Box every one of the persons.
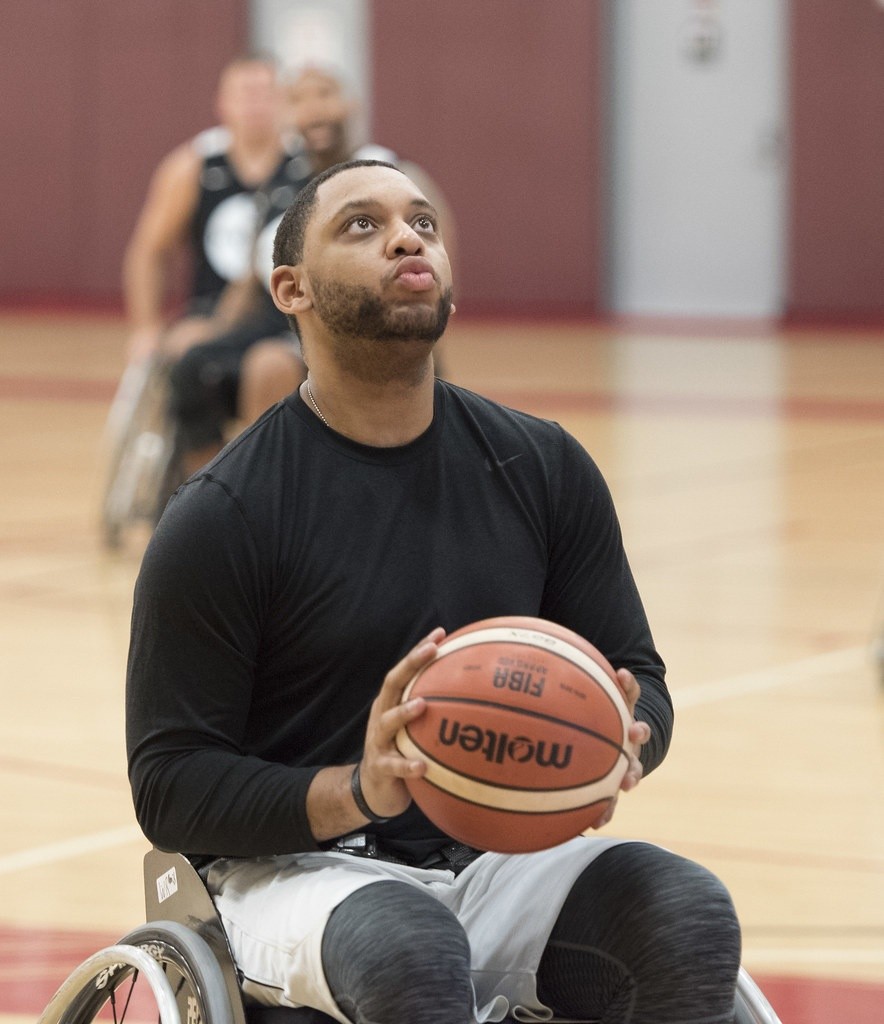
[123,154,757,1023]
[123,42,363,500]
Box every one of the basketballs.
[394,614,634,855]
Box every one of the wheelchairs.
[29,848,783,1023]
[95,352,195,556]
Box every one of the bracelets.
[349,764,396,826]
